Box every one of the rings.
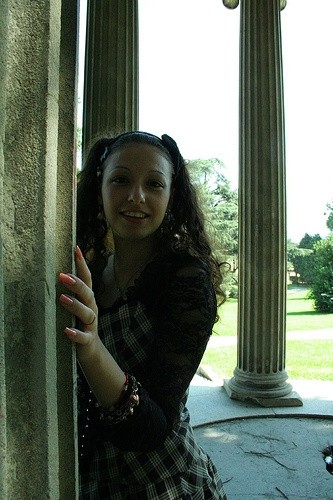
[84,313,96,326]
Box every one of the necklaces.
[113,252,154,300]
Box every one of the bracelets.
[96,372,140,424]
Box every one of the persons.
[59,131,228,500]
